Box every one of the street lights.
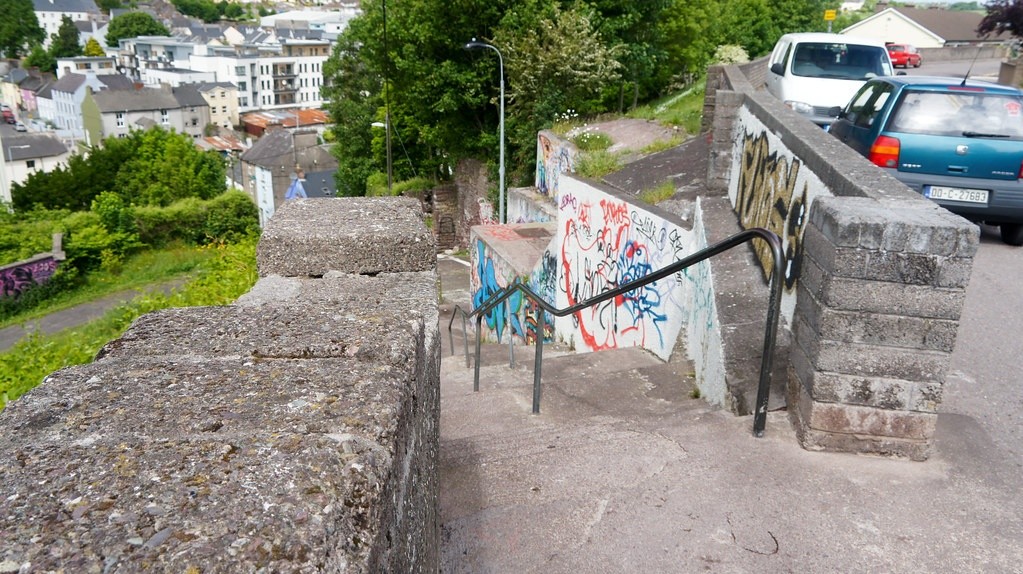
[466,42,505,224]
[8,144,31,190]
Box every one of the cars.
[885,43,922,68]
[1,104,26,132]
[828,76,1023,246]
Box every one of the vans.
[763,32,897,132]
